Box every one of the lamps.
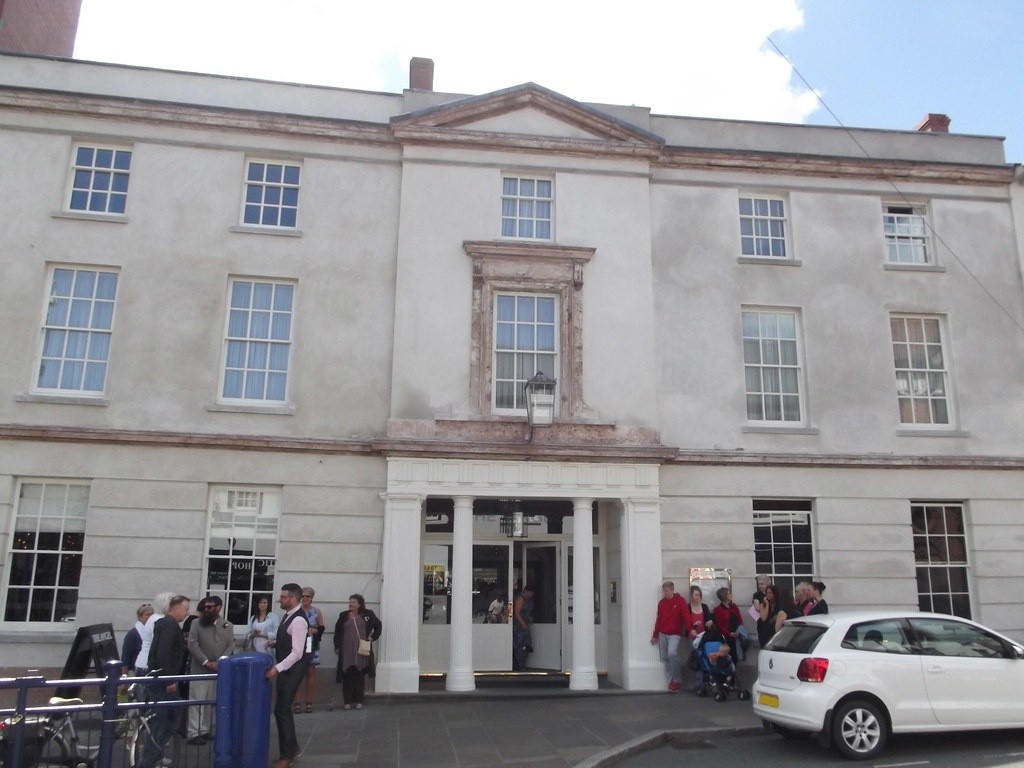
[524,370,557,442]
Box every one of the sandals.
[305,703,312,713]
[293,702,302,714]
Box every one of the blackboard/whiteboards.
[52,624,123,720]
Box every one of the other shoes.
[292,749,302,759]
[356,703,362,709]
[136,737,144,749]
[669,678,680,690]
[188,736,207,745]
[201,734,215,740]
[176,729,187,738]
[155,757,172,765]
[124,736,133,751]
[270,757,295,768]
[164,742,169,747]
[345,704,351,709]
[667,687,677,695]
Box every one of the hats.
[812,581,826,597]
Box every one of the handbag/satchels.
[686,650,699,671]
[306,628,312,653]
[520,633,534,653]
[748,604,761,622]
[358,639,371,656]
[244,632,256,652]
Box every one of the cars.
[748,613,1024,757]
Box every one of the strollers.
[694,624,754,705]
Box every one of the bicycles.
[0,669,181,768]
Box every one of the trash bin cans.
[214,651,274,768]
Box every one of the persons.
[121,604,154,751]
[135,592,191,768]
[188,596,236,745]
[712,587,743,658]
[513,585,536,670]
[650,581,692,692]
[246,596,279,655]
[334,594,382,712]
[488,593,505,623]
[294,587,325,713]
[686,585,713,638]
[710,644,730,660]
[753,575,829,649]
[266,583,310,768]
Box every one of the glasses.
[143,603,151,608]
[202,604,216,610]
[279,595,294,599]
[302,595,310,599]
